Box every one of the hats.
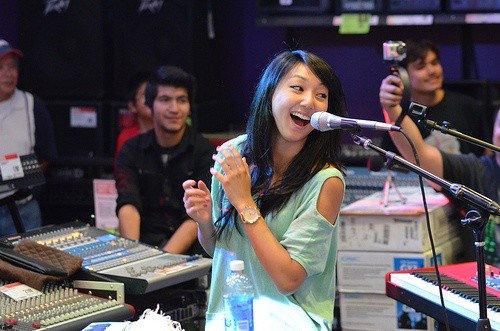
[0,40,22,64]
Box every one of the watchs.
[239,206,264,225]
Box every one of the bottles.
[222,259,255,331]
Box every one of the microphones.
[310,111,401,132]
[367,156,410,174]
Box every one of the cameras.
[383,40,407,61]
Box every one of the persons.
[114,64,223,260]
[379,72,500,270]
[112,72,156,178]
[183,49,347,331]
[377,38,496,159]
[1,39,59,240]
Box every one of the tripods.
[379,176,407,208]
